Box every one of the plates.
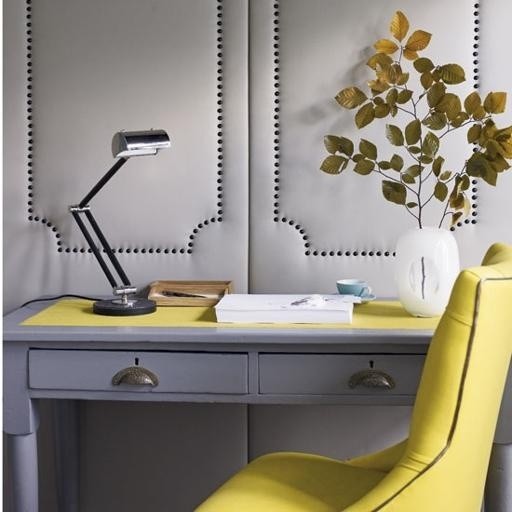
[359,294,377,302]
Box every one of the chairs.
[197,242,512,512]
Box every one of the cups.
[336,278,371,298]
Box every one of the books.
[213,293,354,324]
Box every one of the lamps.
[68,129,171,316]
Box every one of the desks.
[3,298,512,511]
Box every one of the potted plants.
[320,9,512,320]
